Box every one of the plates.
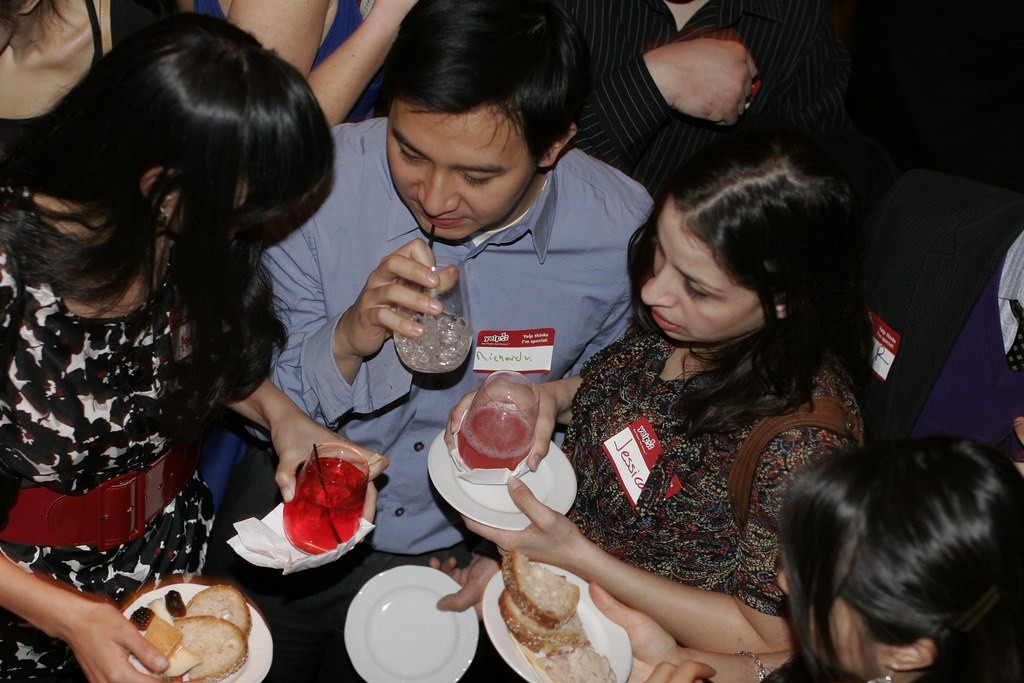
[482,562,633,683]
[122,583,273,683]
[344,565,479,683]
[427,429,577,531]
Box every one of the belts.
[1,426,201,551]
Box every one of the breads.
[174,618,248,683]
[499,550,579,630]
[513,631,592,655]
[183,585,250,637]
[498,587,584,642]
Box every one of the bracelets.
[734,650,765,682]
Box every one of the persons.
[442,125,864,683]
[1,15,389,683]
[220,0,659,682]
[589,444,1024,683]
[0,0,1023,438]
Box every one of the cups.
[392,257,473,373]
[457,371,540,471]
[283,442,370,556]
